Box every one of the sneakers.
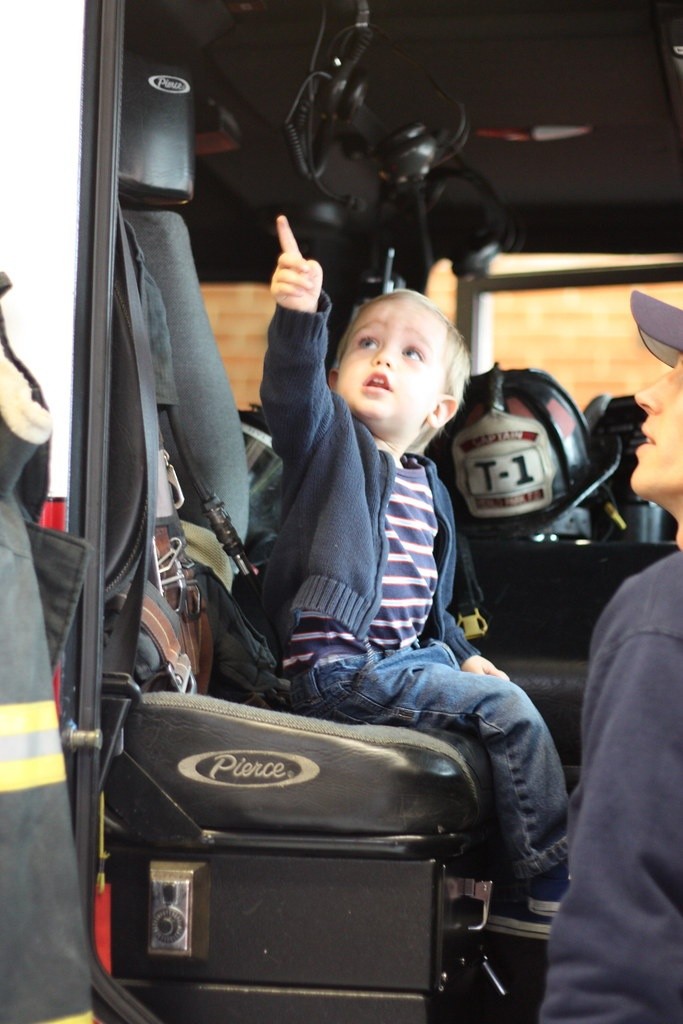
[486,901,554,939]
[529,875,571,918]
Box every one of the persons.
[259,215,571,918]
[534,290,683,1024]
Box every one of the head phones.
[330,25,469,178]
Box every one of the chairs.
[103,194,496,857]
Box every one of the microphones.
[309,94,366,214]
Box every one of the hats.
[630,290,683,368]
